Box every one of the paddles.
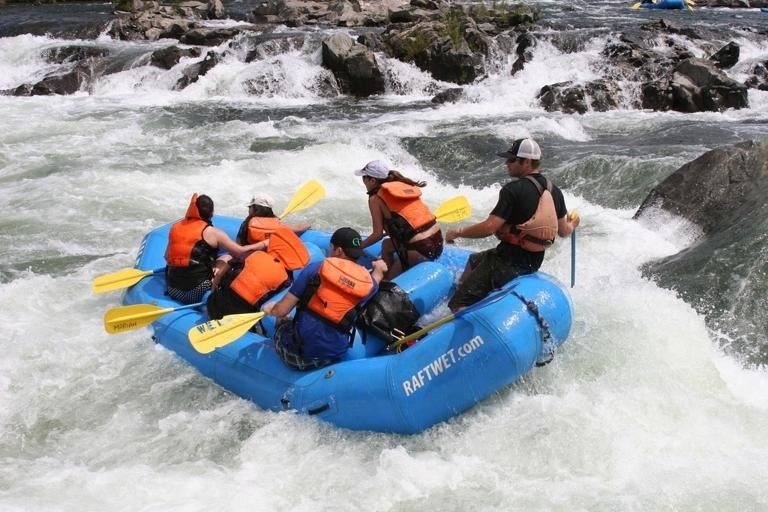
[188,268,374,354]
[385,283,517,353]
[382,196,472,237]
[92,267,166,295]
[105,300,208,333]
[278,179,325,219]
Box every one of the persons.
[234,196,311,270]
[163,194,273,309]
[355,160,444,281]
[205,247,292,322]
[444,134,582,314]
[262,225,389,371]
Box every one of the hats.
[245,197,273,209]
[354,160,390,180]
[330,227,366,258]
[495,138,544,161]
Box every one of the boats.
[122,214,575,433]
[639,0,686,10]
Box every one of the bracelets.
[261,240,266,249]
[457,226,465,238]
